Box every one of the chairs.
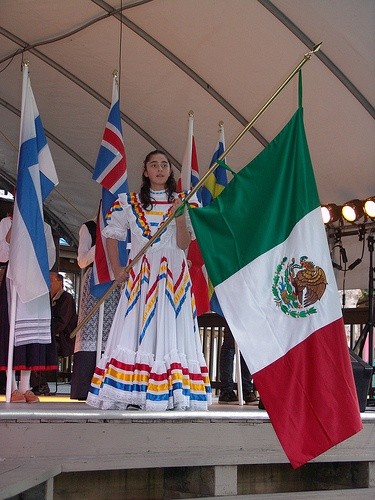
[37,314,78,395]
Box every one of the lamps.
[341,199,364,221]
[363,196,375,218]
[321,203,340,223]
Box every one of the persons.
[84,150,212,411]
[31,273,75,396]
[0,186,57,404]
[218,318,257,405]
[70,197,120,399]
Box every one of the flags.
[6,67,363,470]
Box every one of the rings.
[177,202,179,204]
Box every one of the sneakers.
[10,390,26,402]
[24,390,39,403]
[243,392,259,404]
[218,391,245,405]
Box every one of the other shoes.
[127,404,140,409]
[32,383,50,395]
